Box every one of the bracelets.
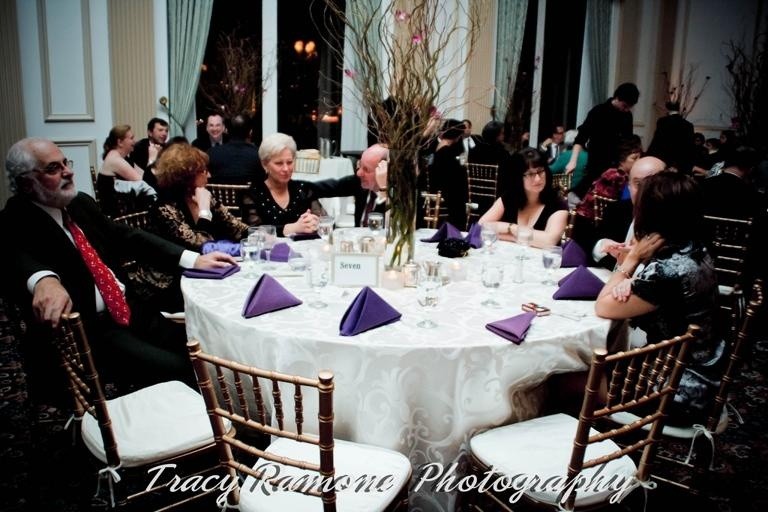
[617,266,630,278]
[508,223,514,232]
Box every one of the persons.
[243,132,335,236]
[424,120,466,186]
[646,100,694,168]
[565,82,640,173]
[460,119,481,149]
[678,161,723,184]
[190,112,231,151]
[721,130,736,143]
[207,114,267,185]
[125,118,169,169]
[575,147,640,229]
[707,144,767,224]
[132,144,273,319]
[368,91,423,143]
[317,143,390,228]
[557,170,729,429]
[539,123,565,164]
[144,136,190,188]
[546,129,588,192]
[504,123,529,155]
[97,125,143,199]
[468,121,511,166]
[478,148,569,249]
[575,157,666,268]
[694,132,708,154]
[0,137,238,394]
[705,138,721,154]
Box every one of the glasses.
[356,160,374,173]
[523,169,544,177]
[21,160,74,175]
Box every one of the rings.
[646,233,650,239]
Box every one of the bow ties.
[154,140,165,146]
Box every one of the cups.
[262,224,277,270]
[316,216,333,243]
[306,263,330,310]
[240,238,262,278]
[287,245,310,277]
[416,275,443,330]
[480,263,501,309]
[515,226,535,261]
[481,228,497,257]
[250,227,265,270]
[541,245,562,286]
[367,211,384,237]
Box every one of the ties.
[215,142,220,158]
[362,192,377,227]
[555,145,559,160]
[467,137,471,151]
[59,206,130,326]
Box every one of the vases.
[380,149,421,273]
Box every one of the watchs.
[379,187,386,191]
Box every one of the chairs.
[464,161,499,231]
[552,173,573,210]
[418,191,442,230]
[591,196,615,225]
[696,213,751,310]
[114,212,155,267]
[186,339,413,512]
[205,185,251,220]
[45,311,235,507]
[593,278,763,507]
[457,325,698,511]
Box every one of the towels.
[464,223,484,248]
[338,286,401,335]
[184,264,240,280]
[420,222,463,243]
[243,274,303,319]
[561,240,601,267]
[488,312,537,345]
[262,243,291,262]
[553,268,604,301]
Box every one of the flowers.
[310,1,513,149]
